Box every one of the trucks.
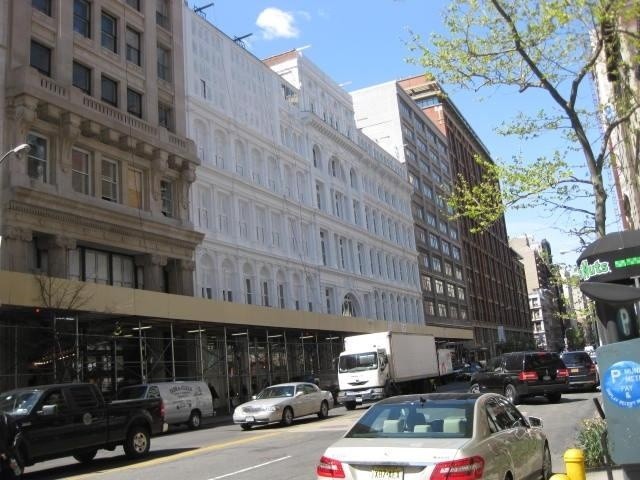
[336,331,439,410]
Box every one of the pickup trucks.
[0,380,166,463]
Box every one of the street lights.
[564,327,573,350]
[2,144,33,167]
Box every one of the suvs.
[469,350,570,406]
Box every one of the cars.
[233,381,335,430]
[463,361,482,381]
[315,391,551,479]
[559,351,599,391]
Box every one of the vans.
[110,380,215,433]
